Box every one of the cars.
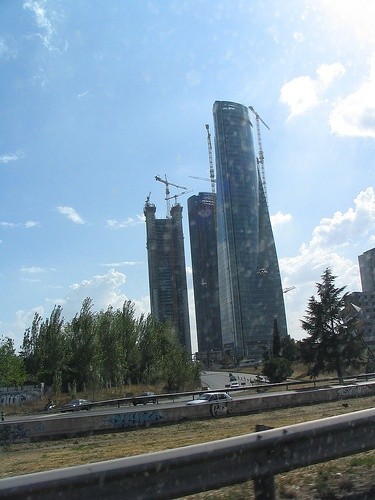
[186,393,232,405]
[61,398,93,413]
[132,391,159,406]
[199,387,210,397]
[230,381,240,387]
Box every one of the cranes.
[144,174,188,220]
[188,123,217,193]
[248,104,270,210]
